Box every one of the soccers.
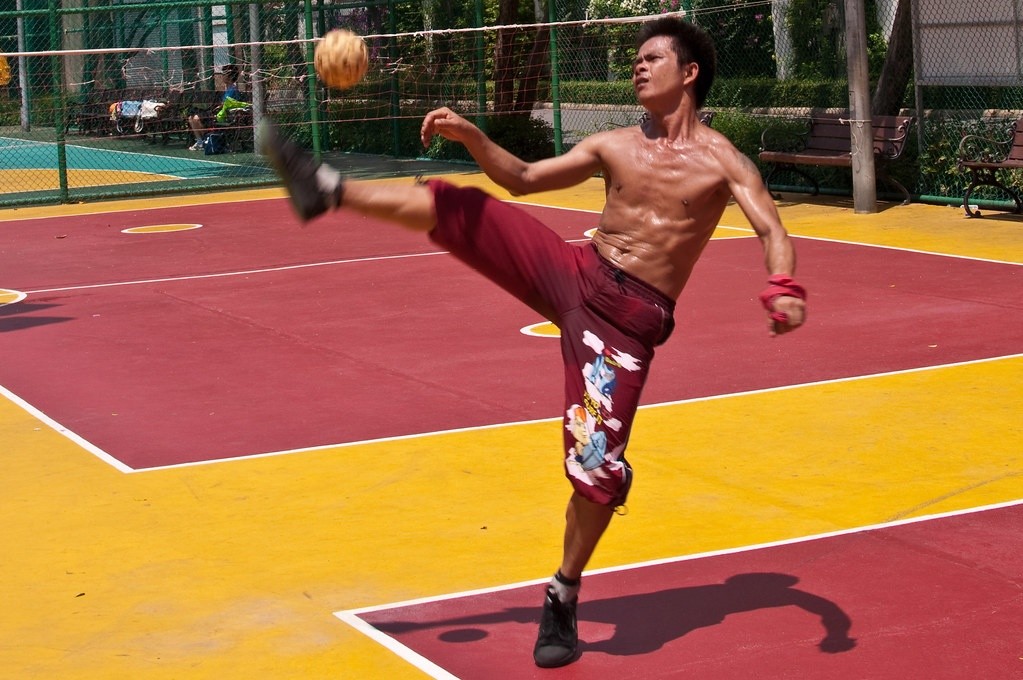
[314,28,370,88]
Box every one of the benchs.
[755,113,914,205]
[954,117,1023,216]
[64,87,270,152]
[597,111,717,177]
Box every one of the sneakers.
[531,586,578,667]
[258,116,329,220]
[189,140,206,152]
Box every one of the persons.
[188,64,241,151]
[258,17,807,669]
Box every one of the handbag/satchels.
[108,98,166,133]
[203,133,225,154]
